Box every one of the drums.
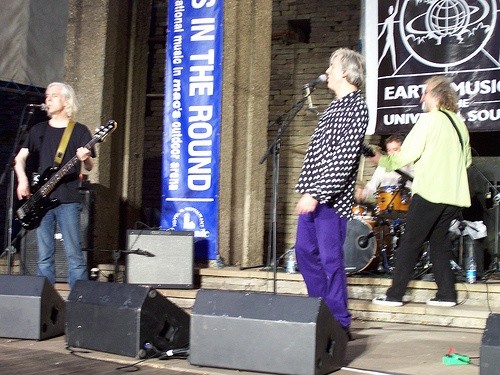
[352,204,368,215]
[374,186,412,219]
[338,218,376,275]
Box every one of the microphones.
[302,73,327,90]
[27,103,48,110]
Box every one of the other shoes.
[426,297,457,306]
[371,294,404,307]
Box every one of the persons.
[295,48,369,341]
[354,76,472,307]
[13,82,96,289]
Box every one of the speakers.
[65,279,191,358]
[478,312,500,375]
[126,229,193,288]
[188,287,348,375]
[0,275,67,339]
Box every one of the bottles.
[466,257,477,284]
[116,265,127,284]
[285,250,296,274]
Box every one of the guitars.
[14,119,118,229]
[360,143,413,182]
